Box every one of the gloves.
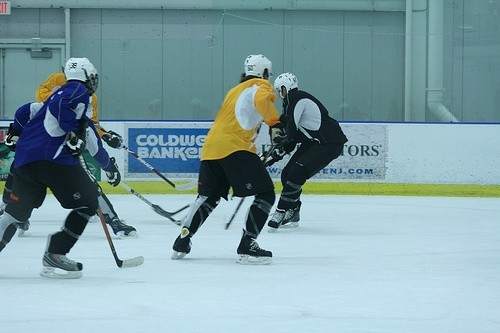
[269,123,289,144]
[103,159,122,186]
[102,130,123,148]
[4,122,22,152]
[260,145,286,167]
[64,131,85,158]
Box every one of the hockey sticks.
[121,143,199,190]
[97,205,145,268]
[153,158,277,226]
[224,144,275,230]
[119,181,190,218]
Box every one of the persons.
[5,73,139,238]
[267,73,350,233]
[171,54,285,264]
[0,57,122,278]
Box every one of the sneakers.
[236,229,272,264]
[266,208,288,233]
[281,201,302,228]
[16,220,30,237]
[171,226,193,259]
[40,234,83,279]
[103,213,137,240]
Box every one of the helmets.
[273,72,298,93]
[244,54,273,78]
[64,58,100,96]
